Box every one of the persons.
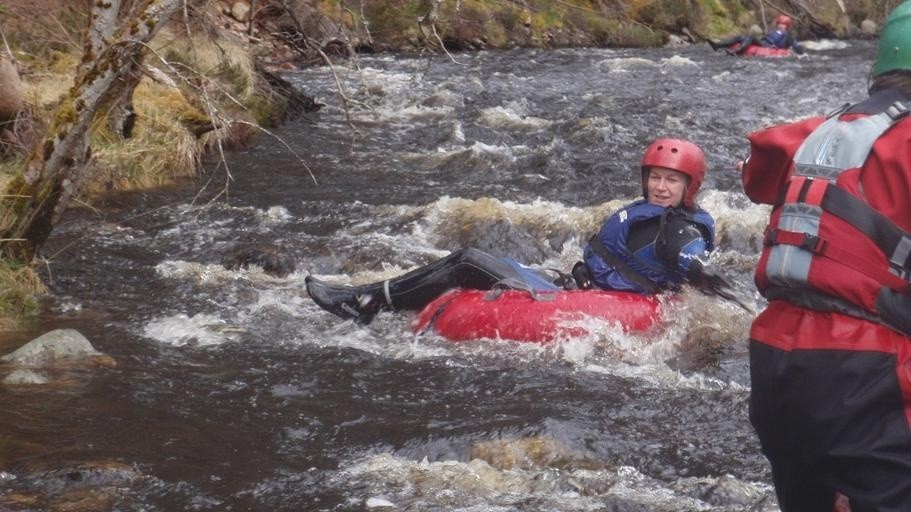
[707,14,806,57]
[304,137,736,325]
[738,1,911,512]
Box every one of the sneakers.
[707,38,719,51]
[305,274,381,327]
[723,48,737,57]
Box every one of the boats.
[729,42,792,59]
[405,287,699,348]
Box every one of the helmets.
[641,139,707,209]
[871,2,910,76]
[776,14,792,27]
